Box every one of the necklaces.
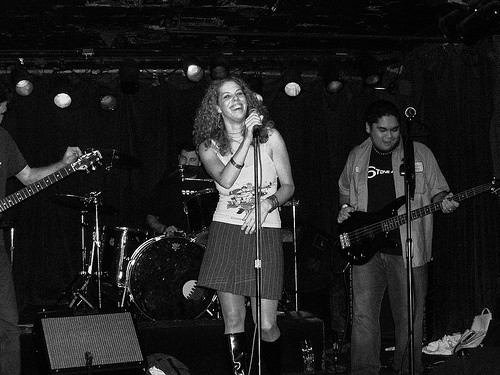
[373,145,397,155]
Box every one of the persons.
[145,145,220,240]
[0,82,82,375]
[192,80,295,375]
[336,98,461,375]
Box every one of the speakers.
[29,306,148,375]
[431,348,500,375]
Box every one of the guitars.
[0,148,103,215]
[334,178,500,266]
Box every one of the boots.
[223,332,248,375]
[261,333,281,375]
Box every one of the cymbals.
[45,193,120,215]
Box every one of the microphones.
[404,106,417,118]
[247,108,260,138]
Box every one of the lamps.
[182,56,205,82]
[374,73,386,90]
[282,63,302,97]
[210,58,231,80]
[318,62,345,93]
[12,64,33,96]
[54,70,72,108]
[95,74,119,114]
[359,66,380,87]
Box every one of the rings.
[448,208,452,211]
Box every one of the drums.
[189,229,249,311]
[96,225,153,289]
[182,187,220,246]
[125,232,217,325]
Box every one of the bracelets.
[230,158,245,169]
[267,195,278,213]
